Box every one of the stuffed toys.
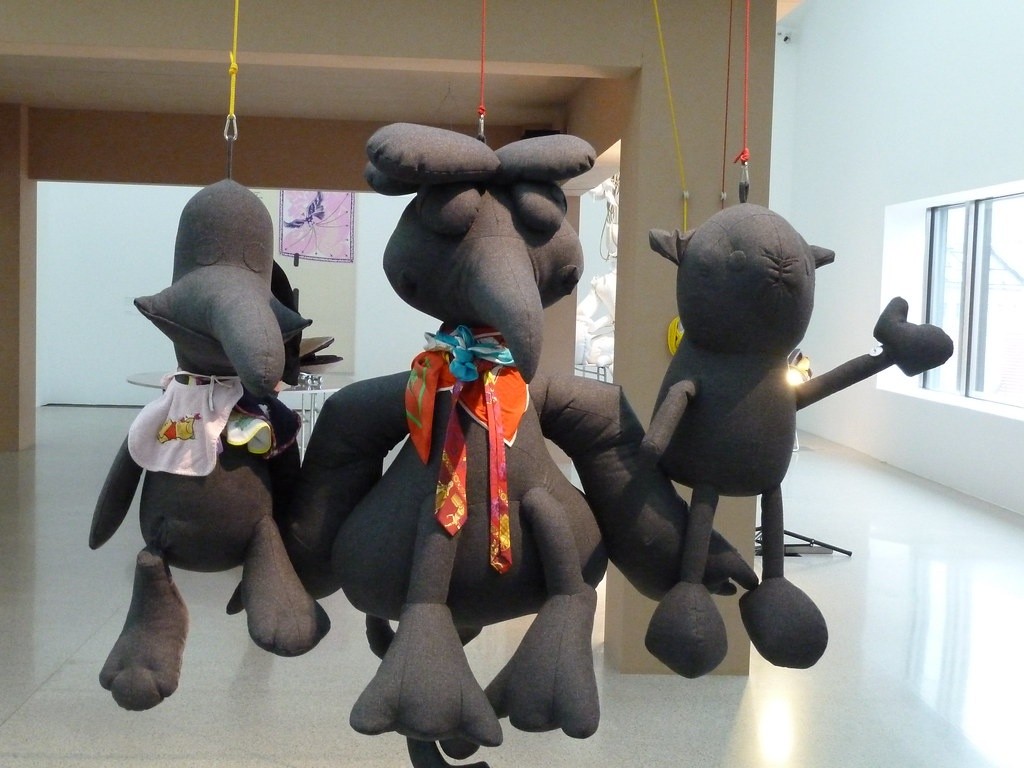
[87,176,346,712]
[637,202,954,680]
[225,123,759,768]
